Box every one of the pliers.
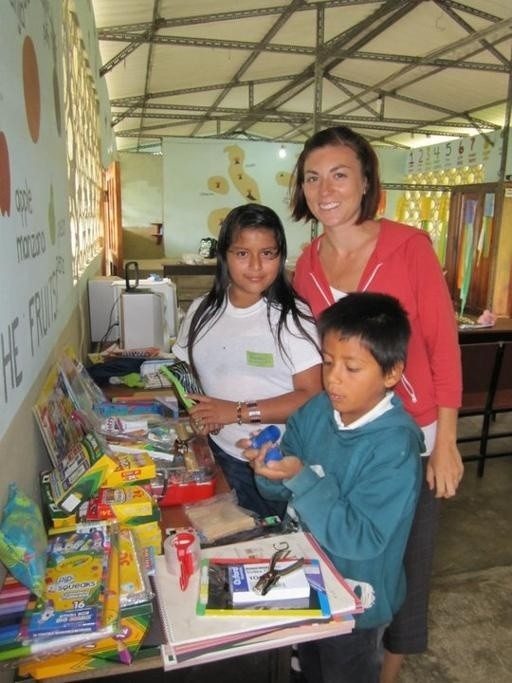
[253,549,304,595]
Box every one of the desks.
[453,309,512,342]
[11,363,291,682]
[160,259,218,279]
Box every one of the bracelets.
[236,399,242,424]
[248,400,262,424]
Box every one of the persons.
[237,292,422,683]
[292,125,464,682]
[173,204,321,515]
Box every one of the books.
[152,531,364,671]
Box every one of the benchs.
[450,341,512,478]
